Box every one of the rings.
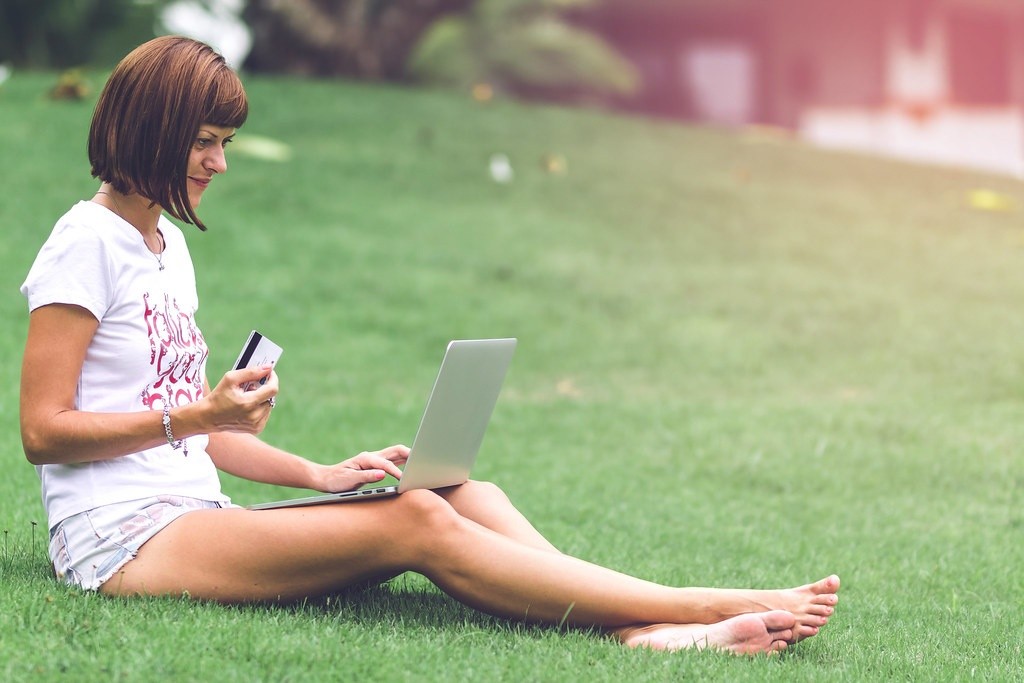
[267,398,275,408]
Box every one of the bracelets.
[163,405,188,457]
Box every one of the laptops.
[246,338,517,509]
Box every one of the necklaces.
[95,191,165,271]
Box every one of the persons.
[18,34,840,658]
